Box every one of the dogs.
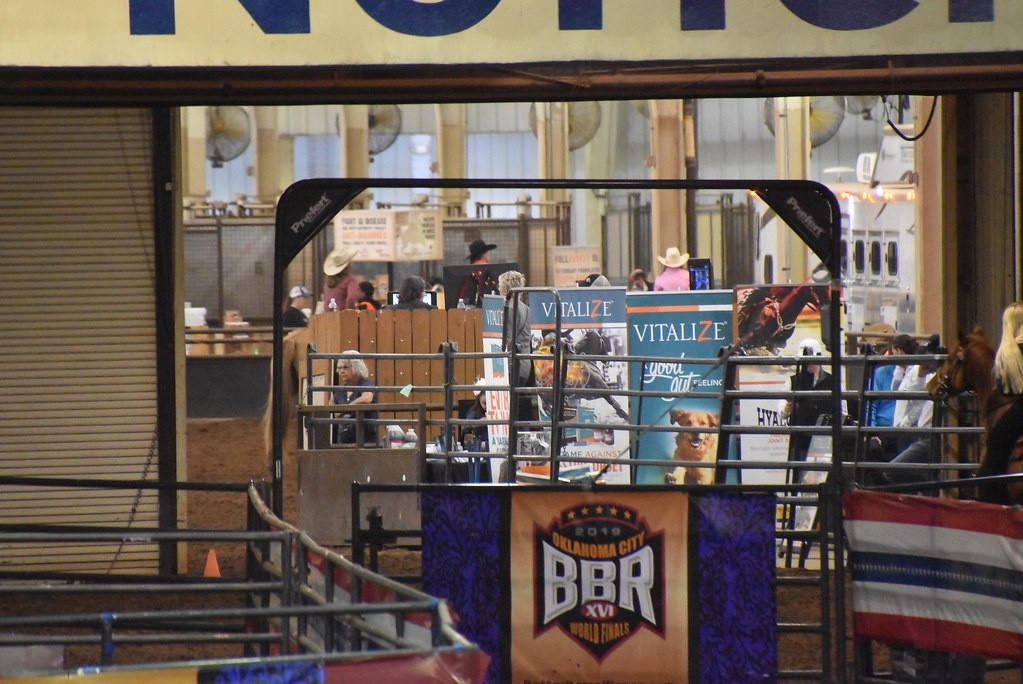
[664,409,720,485]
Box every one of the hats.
[289,286,314,299]
[575,273,611,287]
[324,247,359,275]
[657,246,690,268]
[798,338,822,356]
[465,239,498,260]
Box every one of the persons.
[466,271,498,308]
[280,246,440,331]
[781,299,1023,502]
[329,350,379,446]
[579,247,690,294]
[467,239,536,449]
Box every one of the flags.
[482,287,743,483]
[418,482,776,683]
[840,486,1022,665]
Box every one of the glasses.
[338,366,352,370]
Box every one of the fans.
[764,95,882,155]
[335,102,402,163]
[207,106,251,168]
[530,101,603,150]
